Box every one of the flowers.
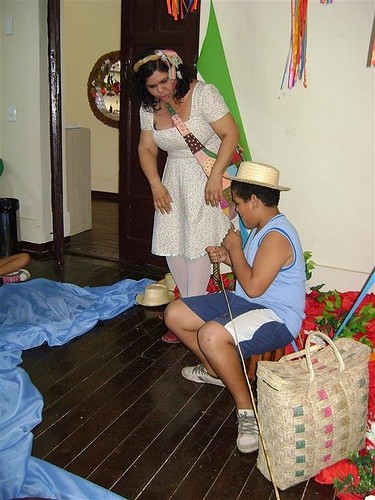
[91,73,120,97]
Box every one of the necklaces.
[164,104,180,119]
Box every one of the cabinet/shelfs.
[65,129,92,236]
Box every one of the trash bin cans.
[0,198,19,257]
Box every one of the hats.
[136,284,176,306]
[156,273,177,291]
[222,161,292,191]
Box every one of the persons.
[165,161,305,453]
[0,253,31,287]
[128,45,245,343]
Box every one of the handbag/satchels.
[256,331,372,491]
[192,143,243,231]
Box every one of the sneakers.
[181,364,226,387]
[236,409,260,453]
[0,269,30,284]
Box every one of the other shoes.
[162,330,181,343]
[159,311,164,320]
